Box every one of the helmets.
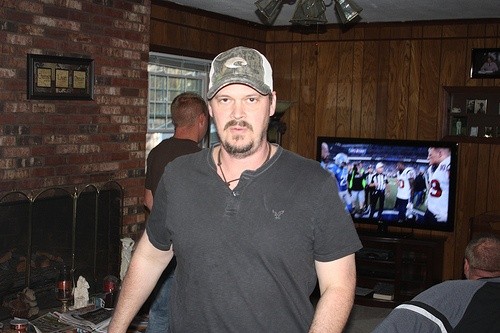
[335,153,348,167]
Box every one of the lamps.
[255,0,363,25]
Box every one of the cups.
[62,301,68,313]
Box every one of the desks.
[0,299,150,333]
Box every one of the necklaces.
[218,142,271,185]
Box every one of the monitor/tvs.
[316,136,458,240]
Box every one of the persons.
[476,102,485,114]
[481,57,498,71]
[369,235,500,333]
[106,47,364,333]
[320,142,451,224]
[455,118,462,136]
[143,90,213,211]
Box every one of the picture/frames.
[442,85,500,145]
[470,48,500,79]
[27,53,94,100]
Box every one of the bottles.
[58,265,71,299]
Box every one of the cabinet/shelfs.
[354,227,448,308]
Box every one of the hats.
[376,162,384,168]
[207,45,273,98]
